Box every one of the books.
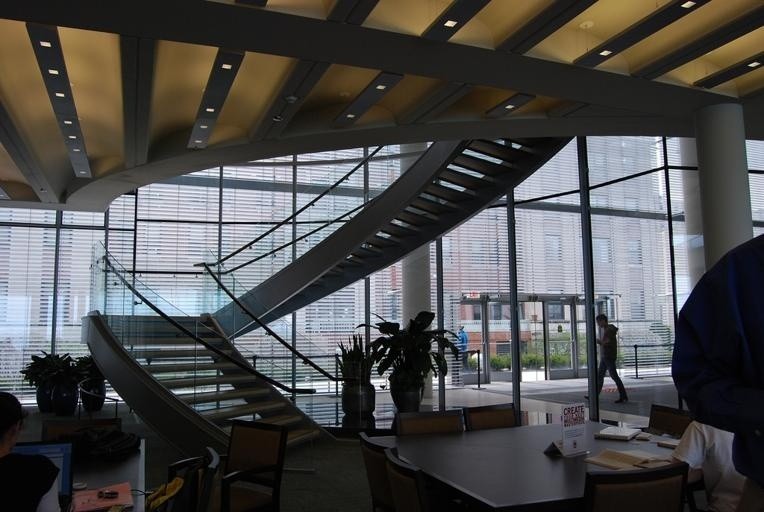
[583,424,680,472]
[73,481,134,512]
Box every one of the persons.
[584,315,629,404]
[671,419,748,510]
[0,391,61,512]
[458,327,468,370]
[670,235,764,510]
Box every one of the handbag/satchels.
[51,424,141,465]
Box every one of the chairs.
[41,416,288,512]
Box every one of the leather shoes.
[584,396,589,400]
[615,396,628,403]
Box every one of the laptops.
[11,440,74,512]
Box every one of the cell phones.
[98,489,119,499]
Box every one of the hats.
[0,392,29,431]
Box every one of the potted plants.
[336,334,376,416]
[19,349,107,417]
[356,310,461,413]
